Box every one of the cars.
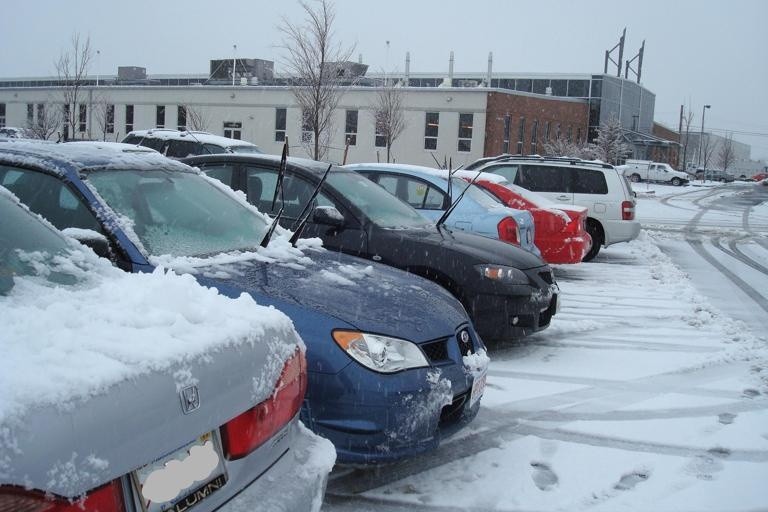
[697,169,735,183]
[173,153,562,351]
[0,183,338,512]
[751,173,768,181]
[1,138,491,470]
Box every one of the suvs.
[119,128,265,158]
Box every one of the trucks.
[684,161,700,179]
[625,159,689,186]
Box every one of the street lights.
[698,104,711,166]
